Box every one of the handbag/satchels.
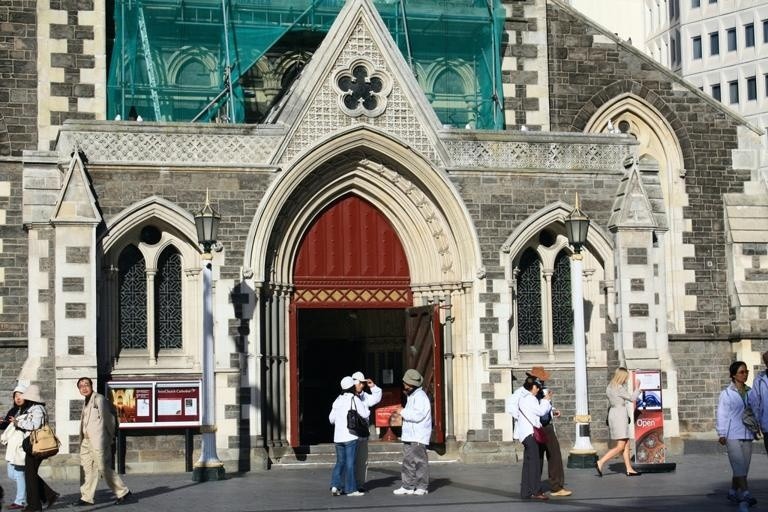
[346,396,370,437]
[533,426,550,444]
[29,407,63,458]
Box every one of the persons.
[593,366,644,477]
[66,377,133,507]
[351,370,383,492]
[127,105,139,122]
[0,382,29,510]
[525,365,571,496]
[328,376,371,498]
[714,360,758,510]
[514,376,554,502]
[747,350,768,456]
[8,383,60,510]
[392,368,432,496]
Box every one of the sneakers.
[21,503,43,511]
[8,503,24,508]
[523,494,549,500]
[393,486,415,495]
[414,488,429,495]
[743,496,757,505]
[331,487,341,496]
[46,492,60,508]
[726,493,743,504]
[346,491,365,497]
[550,488,572,496]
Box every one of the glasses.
[737,370,749,374]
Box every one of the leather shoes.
[115,490,132,505]
[627,472,642,476]
[594,460,602,477]
[72,499,95,506]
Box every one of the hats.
[340,376,360,390]
[402,369,425,387]
[352,371,368,382]
[526,365,550,381]
[21,384,45,404]
[12,385,26,394]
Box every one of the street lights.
[192,186,226,479]
[566,193,599,469]
